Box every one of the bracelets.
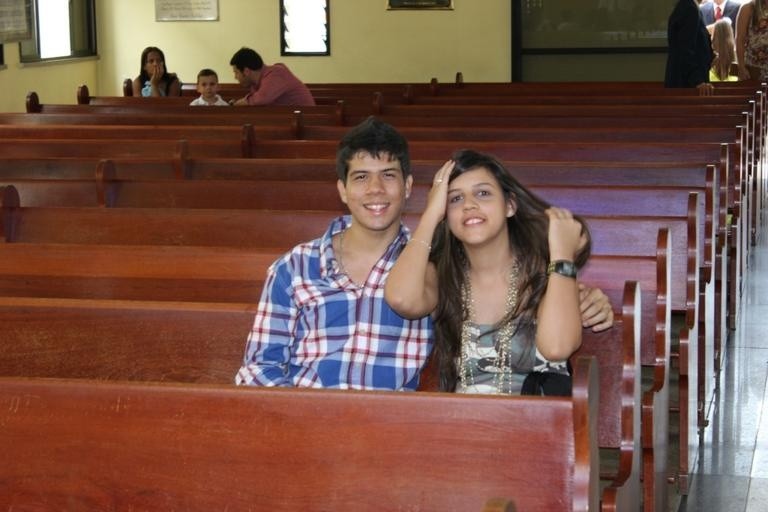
[405,237,433,252]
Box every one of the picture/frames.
[154,0,220,23]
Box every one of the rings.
[435,177,443,184]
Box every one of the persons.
[665,0,717,97]
[708,19,741,83]
[698,0,741,40]
[227,46,317,106]
[735,0,768,82]
[235,115,614,396]
[133,45,180,96]
[383,148,590,397]
[189,67,230,106]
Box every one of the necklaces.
[458,253,523,396]
[338,227,365,287]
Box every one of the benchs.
[1,73,766,512]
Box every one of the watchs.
[546,258,578,280]
